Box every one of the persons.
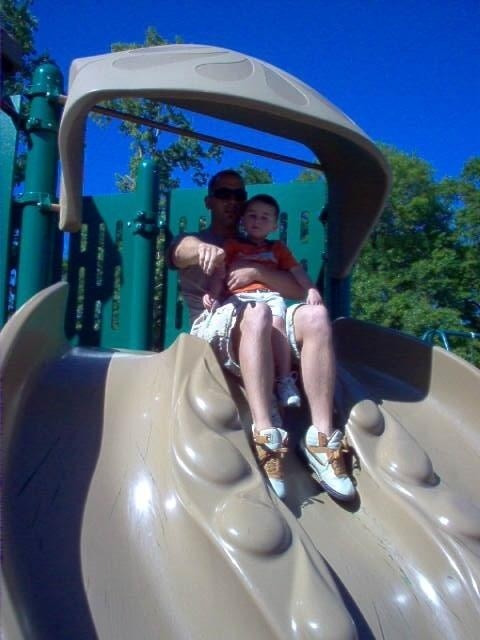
[167,170,358,502]
[201,194,326,410]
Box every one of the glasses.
[210,188,248,203]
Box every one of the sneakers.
[294,424,357,502]
[249,422,290,501]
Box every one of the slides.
[0,282,479,639]
